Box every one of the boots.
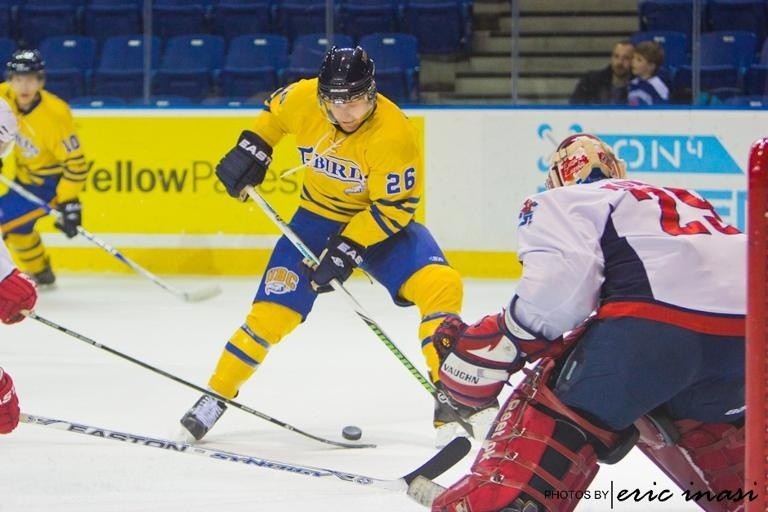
[174,390,228,443]
[31,267,57,292]
[431,379,501,449]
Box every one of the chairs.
[628,0,768,107]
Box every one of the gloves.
[1,264,38,326]
[0,370,21,436]
[53,196,83,239]
[300,233,367,296]
[218,128,273,203]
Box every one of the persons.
[628,42,672,107]
[570,39,638,105]
[180,48,498,441]
[0,238,36,433]
[432,135,750,512]
[1,51,85,290]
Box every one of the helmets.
[5,48,45,73]
[317,45,376,104]
[547,132,623,188]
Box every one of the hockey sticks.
[242,184,497,444]
[15,309,378,447]
[0,173,221,305]
[19,409,472,489]
